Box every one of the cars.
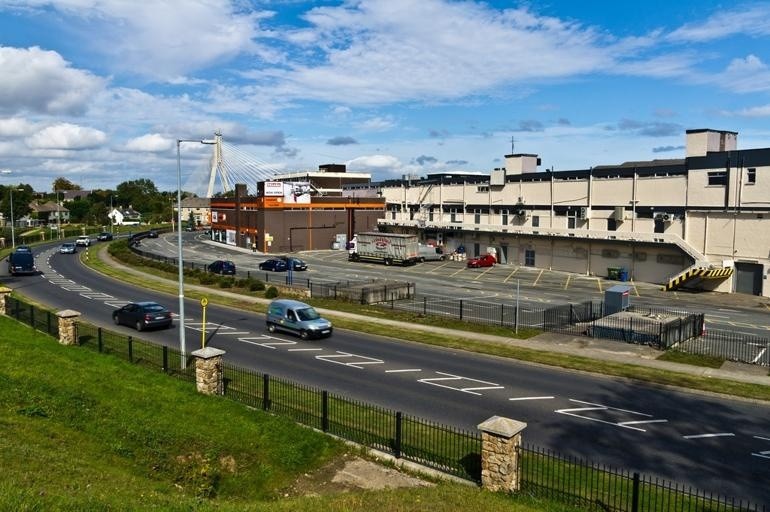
[186,225,196,232]
[112,301,172,332]
[147,232,159,238]
[258,255,308,272]
[208,260,236,275]
[468,254,497,268]
[60,243,76,254]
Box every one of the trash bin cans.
[620,271,628,282]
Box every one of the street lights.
[10,188,119,249]
[176,139,259,374]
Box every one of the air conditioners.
[663,213,674,222]
[519,209,531,221]
[580,207,590,220]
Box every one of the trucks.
[347,230,420,266]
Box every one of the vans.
[5,247,36,275]
[266,299,333,340]
[419,246,445,262]
